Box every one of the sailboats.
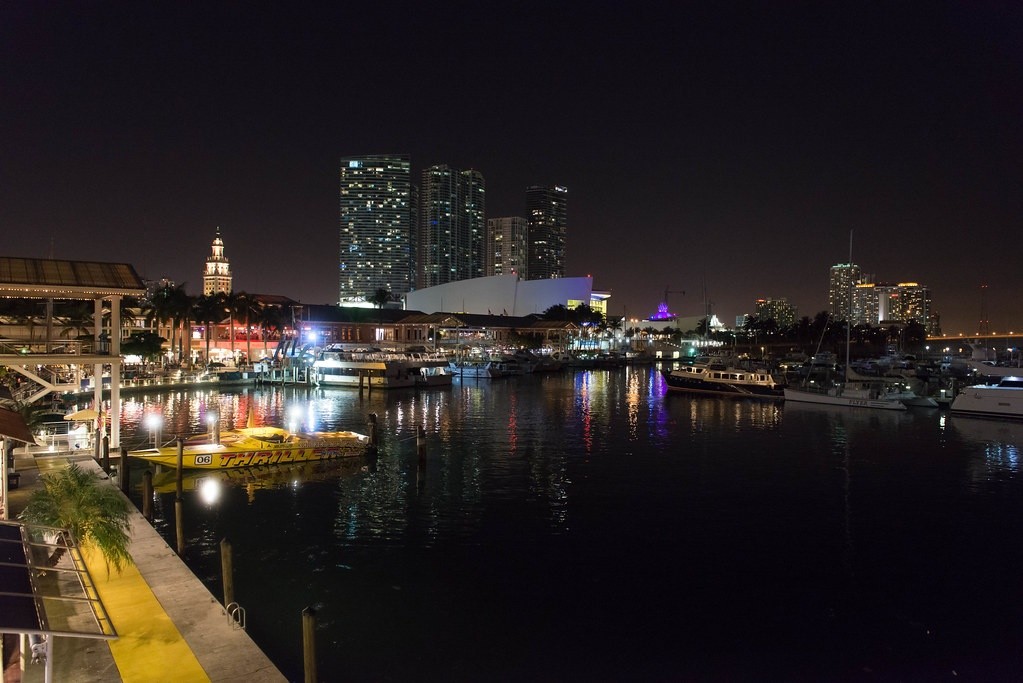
[783,230,907,412]
[658,268,789,400]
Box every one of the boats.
[311,349,458,389]
[449,349,562,380]
[133,425,372,470]
[949,377,1023,423]
